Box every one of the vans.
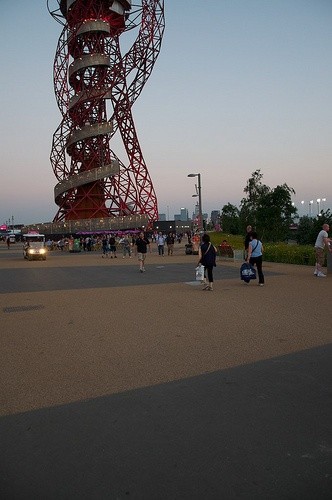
[9,234,16,242]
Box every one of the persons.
[121,235,133,258]
[108,233,117,258]
[314,224,332,277]
[156,232,165,256]
[135,232,150,273]
[198,234,217,291]
[245,225,259,266]
[152,230,185,244]
[101,236,109,258]
[246,232,264,287]
[165,231,174,256]
[47,232,139,254]
[6,237,11,250]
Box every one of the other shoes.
[242,282,250,286]
[200,284,208,290]
[260,284,264,287]
[206,287,214,291]
[317,272,327,278]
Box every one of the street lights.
[188,173,203,232]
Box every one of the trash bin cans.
[185,245,192,255]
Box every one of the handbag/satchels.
[194,265,205,281]
[240,263,256,280]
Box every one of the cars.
[22,233,49,262]
